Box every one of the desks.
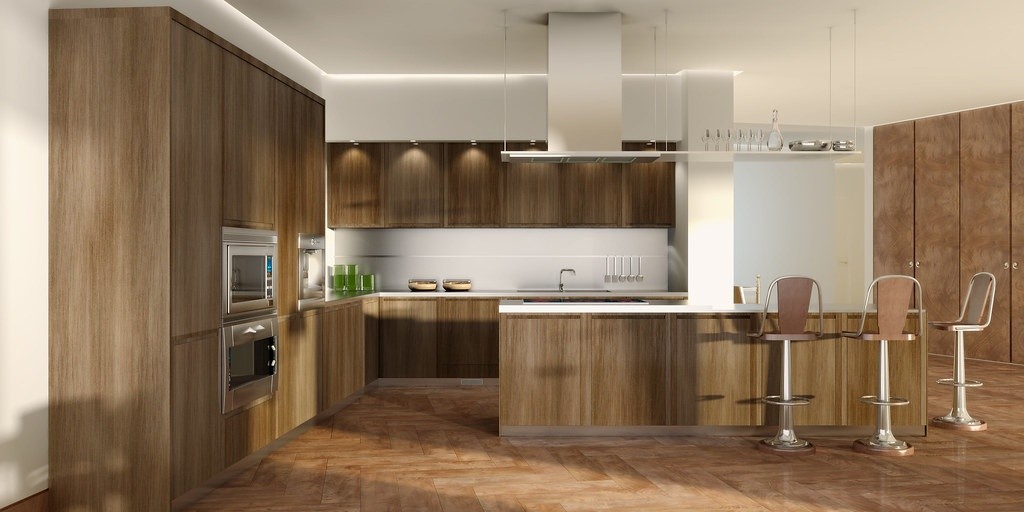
[498,302,930,439]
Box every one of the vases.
[766,108,784,152]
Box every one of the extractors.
[501,12,661,164]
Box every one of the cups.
[744,129,755,150]
[345,264,358,294]
[755,129,765,151]
[333,265,344,292]
[721,128,733,152]
[711,128,721,151]
[733,129,744,150]
[702,129,711,151]
[361,274,374,291]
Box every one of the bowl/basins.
[833,140,855,150]
[408,279,437,291]
[442,279,471,291]
[789,139,831,150]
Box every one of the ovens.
[221,226,278,318]
[222,313,279,414]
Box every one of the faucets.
[559,269,576,291]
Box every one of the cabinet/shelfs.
[443,142,561,229]
[223,36,326,236]
[561,141,676,229]
[871,110,961,358]
[326,141,444,230]
[379,297,500,387]
[46,8,222,512]
[299,299,381,430]
[960,100,1024,366]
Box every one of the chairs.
[745,276,824,457]
[927,272,996,432]
[840,275,924,457]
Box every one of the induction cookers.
[522,298,650,305]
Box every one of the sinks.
[517,290,610,292]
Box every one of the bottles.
[767,109,784,150]
